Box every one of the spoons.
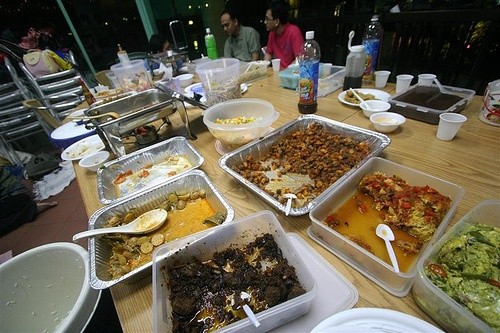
[237,292,261,328]
[283,193,298,217]
[375,222,400,274]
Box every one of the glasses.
[264,16,274,22]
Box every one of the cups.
[271,58,281,73]
[374,71,391,88]
[396,74,437,94]
[197,58,241,102]
[111,59,152,93]
[436,113,467,143]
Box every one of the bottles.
[362,14,383,84]
[298,31,320,113]
[204,27,218,60]
[343,31,365,91]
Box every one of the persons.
[221,9,260,62]
[263,1,304,69]
[142,32,169,53]
[17,23,85,78]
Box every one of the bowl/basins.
[88,115,391,291]
[388,83,476,123]
[174,74,194,86]
[359,99,391,118]
[370,113,406,133]
[413,199,500,333]
[319,62,346,98]
[79,151,110,173]
[308,157,465,290]
[202,97,279,150]
[151,210,319,333]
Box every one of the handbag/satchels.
[22,48,60,77]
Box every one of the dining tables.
[62,67,500,333]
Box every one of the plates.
[338,89,391,107]
[60,134,106,162]
[183,82,248,99]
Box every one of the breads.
[345,90,374,104]
